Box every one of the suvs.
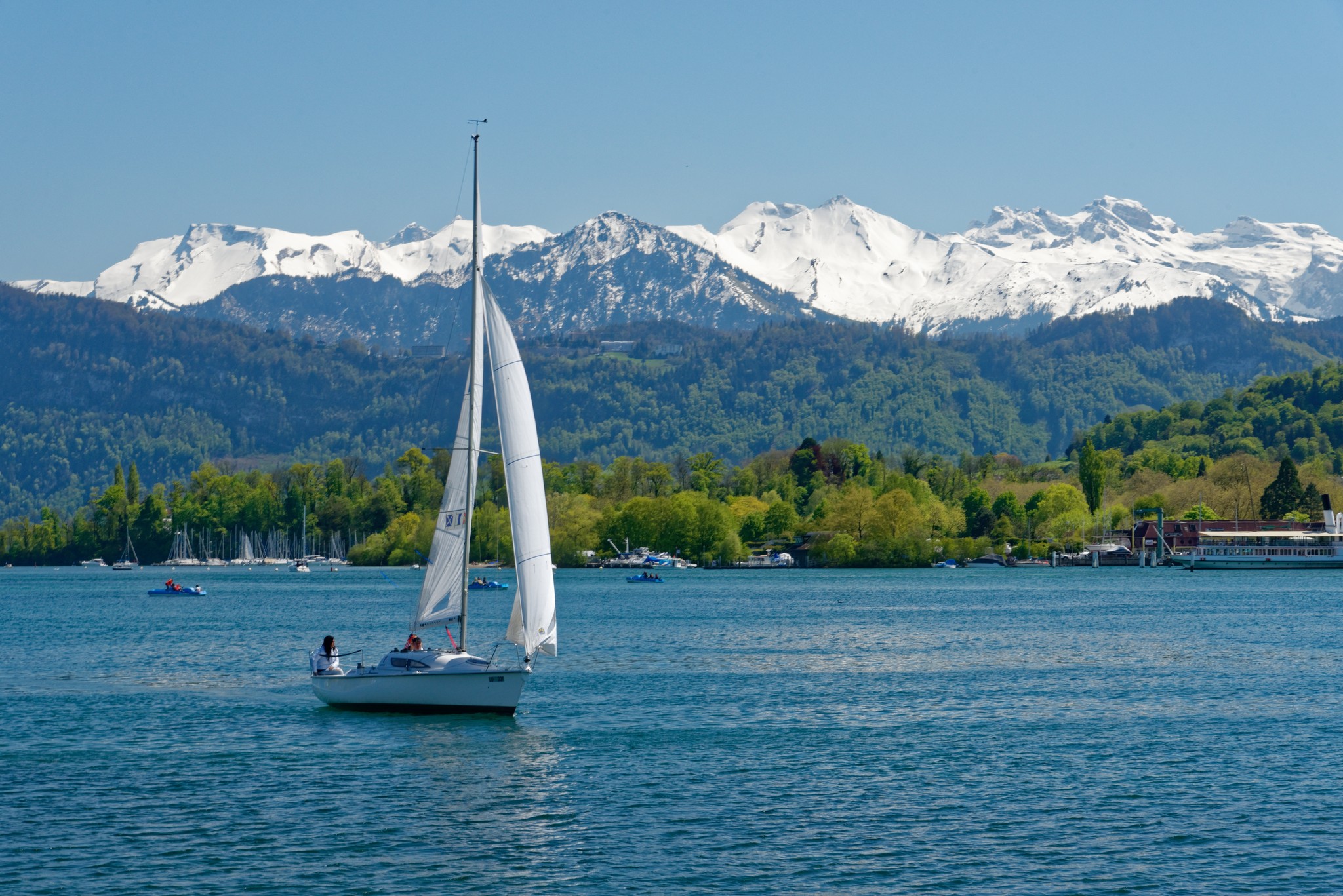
[1056,552,1068,560]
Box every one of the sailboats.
[1079,488,1133,558]
[112,526,140,570]
[287,504,310,572]
[150,521,366,564]
[1014,518,1052,567]
[410,544,422,569]
[306,119,560,712]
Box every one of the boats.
[147,586,207,596]
[468,581,509,590]
[625,575,665,582]
[962,553,1008,568]
[1168,530,1343,568]
[930,558,960,568]
[601,537,697,570]
[79,557,108,567]
[733,549,795,567]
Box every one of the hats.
[412,637,421,644]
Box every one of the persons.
[310,636,344,675]
[472,577,488,586]
[642,571,659,579]
[405,633,427,653]
[165,580,181,591]
[196,585,202,592]
